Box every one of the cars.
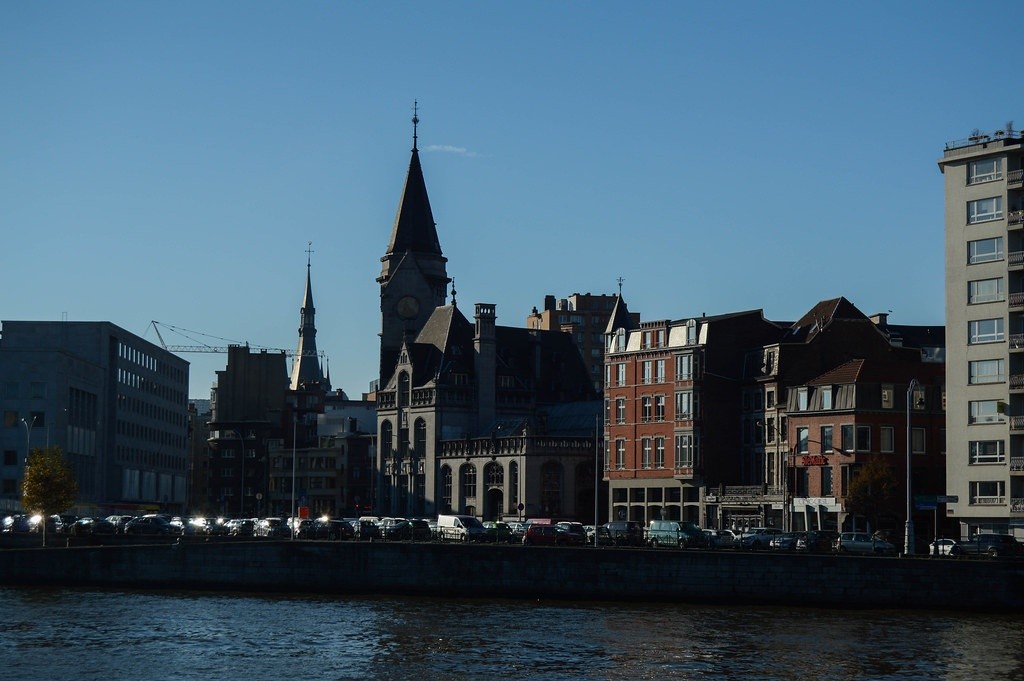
[769,533,808,549]
[839,532,896,557]
[929,538,963,556]
[582,525,609,545]
[949,534,1022,559]
[648,519,707,550]
[601,521,643,544]
[4,514,182,537]
[555,521,586,547]
[521,525,578,547]
[437,514,523,543]
[171,515,431,542]
[733,528,788,548]
[701,527,742,548]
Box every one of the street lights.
[904,379,925,556]
[757,420,852,532]
[21,416,37,514]
[347,416,374,516]
